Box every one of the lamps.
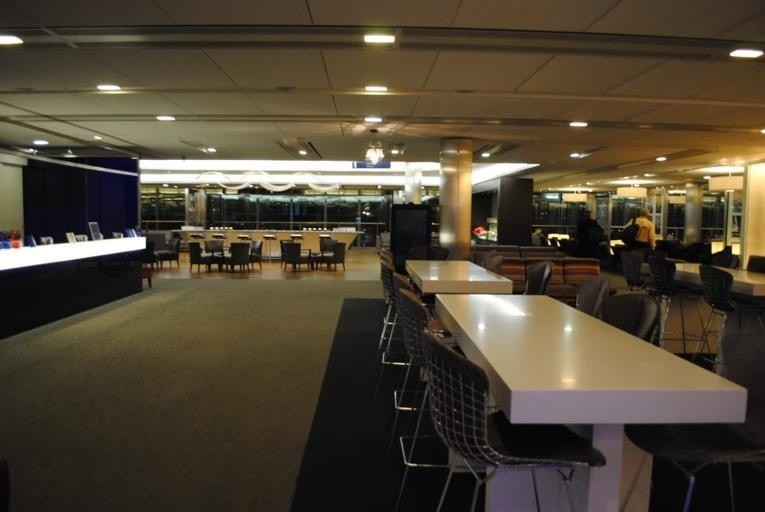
[366,129,384,165]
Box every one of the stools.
[263,234,277,264]
[237,234,250,241]
[212,233,226,240]
[190,233,204,243]
[290,234,303,243]
[319,234,331,240]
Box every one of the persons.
[576,211,604,259]
[622,208,657,255]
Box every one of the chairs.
[397,287,487,511]
[649,255,712,359]
[619,306,765,512]
[711,254,737,268]
[320,243,345,271]
[381,247,393,259]
[563,257,617,303]
[379,252,393,266]
[481,254,526,293]
[280,240,294,267]
[692,264,761,363]
[378,260,401,352]
[525,256,577,302]
[156,239,180,272]
[420,332,606,512]
[283,242,310,272]
[475,245,520,266]
[187,242,214,273]
[621,250,672,321]
[226,243,250,273]
[372,271,444,401]
[523,261,554,295]
[249,241,262,271]
[520,246,561,258]
[601,294,661,346]
[204,241,223,271]
[747,255,765,274]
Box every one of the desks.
[1,236,148,341]
[435,292,748,512]
[405,259,514,294]
[674,263,765,303]
[171,229,365,263]
